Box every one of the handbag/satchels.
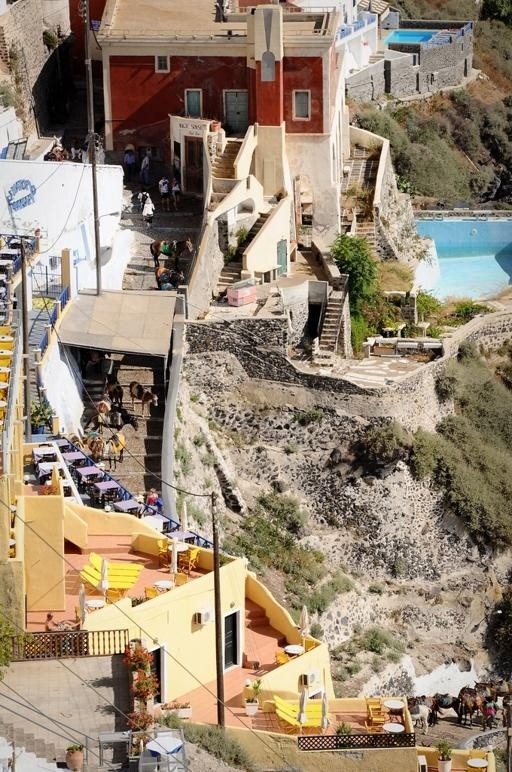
[484,706,495,715]
[156,498,163,508]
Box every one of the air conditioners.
[301,672,317,688]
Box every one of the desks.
[32,438,197,542]
[382,698,406,733]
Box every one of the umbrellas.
[296,685,308,735]
[296,604,309,648]
[319,691,329,735]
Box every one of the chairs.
[78,536,203,605]
[272,694,324,734]
[364,694,386,736]
[465,749,489,772]
[275,638,316,665]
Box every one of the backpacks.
[141,192,148,203]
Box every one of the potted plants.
[435,737,455,772]
[65,743,84,772]
[242,678,263,716]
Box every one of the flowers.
[121,641,191,753]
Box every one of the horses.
[155,267,185,290]
[102,432,126,472]
[84,373,139,434]
[129,381,159,421]
[150,236,194,271]
[406,679,512,735]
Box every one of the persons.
[170,175,181,212]
[123,149,136,177]
[139,152,153,186]
[145,487,159,513]
[137,186,151,213]
[158,174,171,212]
[44,611,60,632]
[478,695,500,731]
[141,197,156,230]
[49,135,84,161]
